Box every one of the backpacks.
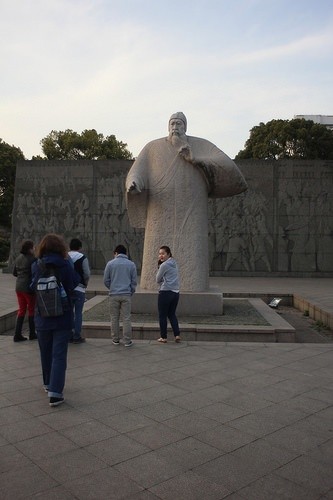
[35,257,72,319]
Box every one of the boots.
[13,316,37,342]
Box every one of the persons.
[125,112,249,294]
[16,171,332,273]
[30,234,82,407]
[102,245,138,346]
[66,238,91,344]
[12,239,38,343]
[154,245,182,341]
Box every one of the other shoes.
[157,335,179,343]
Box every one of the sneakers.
[68,337,81,344]
[49,397,64,407]
[112,340,133,346]
